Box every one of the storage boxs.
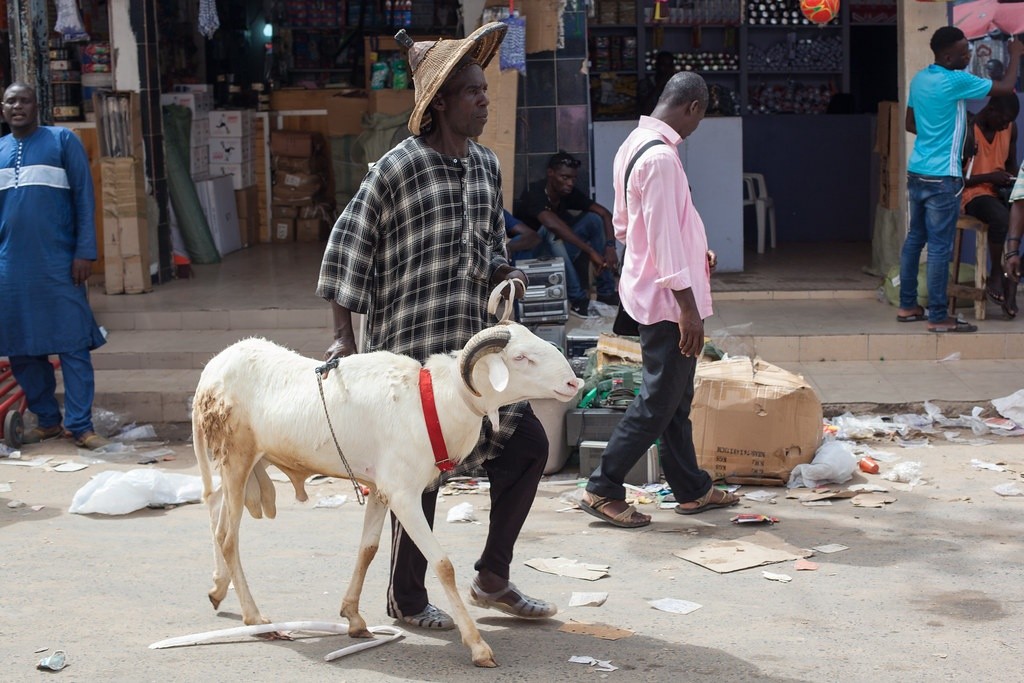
[159,83,324,258]
[471,0,555,216]
[689,352,823,480]
[873,101,899,210]
[92,88,151,295]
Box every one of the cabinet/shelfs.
[586,0,897,118]
[271,1,461,137]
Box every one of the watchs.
[605,239,615,247]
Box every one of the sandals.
[400,603,454,629]
[466,579,558,620]
[579,491,651,528]
[675,484,740,514]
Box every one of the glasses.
[554,159,582,169]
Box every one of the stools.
[947,212,990,321]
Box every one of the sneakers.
[570,302,602,319]
[596,292,620,306]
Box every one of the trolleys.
[0,355,61,450]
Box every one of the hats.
[394,21,508,136]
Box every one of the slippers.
[896,305,929,321]
[929,318,978,333]
[985,279,1006,305]
[1001,300,1017,318]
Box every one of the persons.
[0,83,109,450]
[316,19,622,627]
[896,26,1024,333]
[580,74,739,528]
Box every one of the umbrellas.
[950,0,1023,39]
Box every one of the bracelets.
[1007,237,1020,242]
[1005,247,1019,259]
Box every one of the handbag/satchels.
[613,310,640,336]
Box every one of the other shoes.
[22,425,64,444]
[75,431,111,451]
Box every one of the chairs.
[742,172,777,256]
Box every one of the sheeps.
[189,318,578,669]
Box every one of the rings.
[1004,273,1007,277]
